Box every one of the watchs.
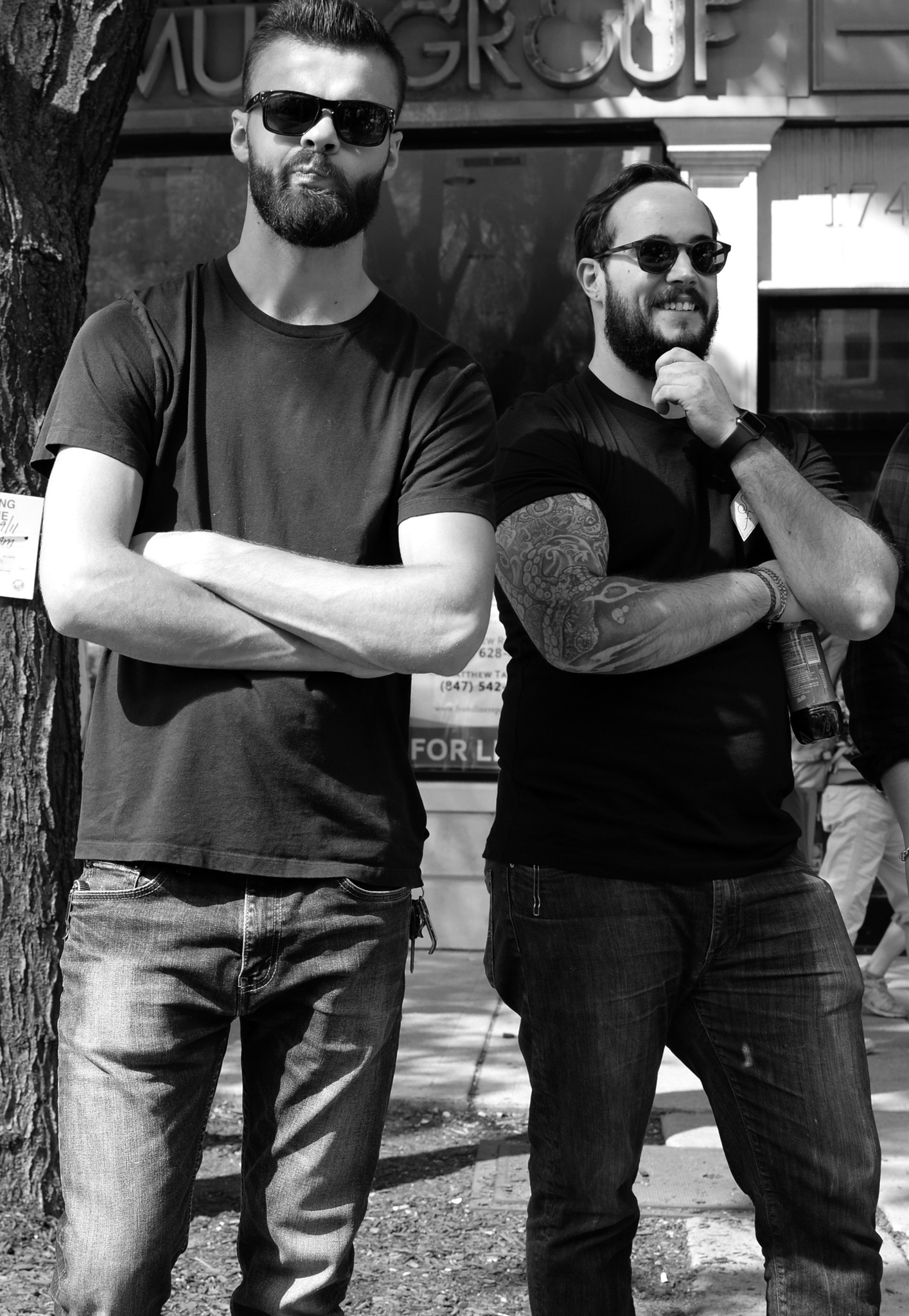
[718,411,765,465]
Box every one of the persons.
[472,162,908,1316]
[29,0,490,1316]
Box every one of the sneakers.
[861,969,909,1017]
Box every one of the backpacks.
[790,630,852,791]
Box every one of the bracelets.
[752,568,788,626]
[743,568,776,620]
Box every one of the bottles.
[774,620,843,745]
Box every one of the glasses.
[245,90,396,148]
[594,237,731,276]
